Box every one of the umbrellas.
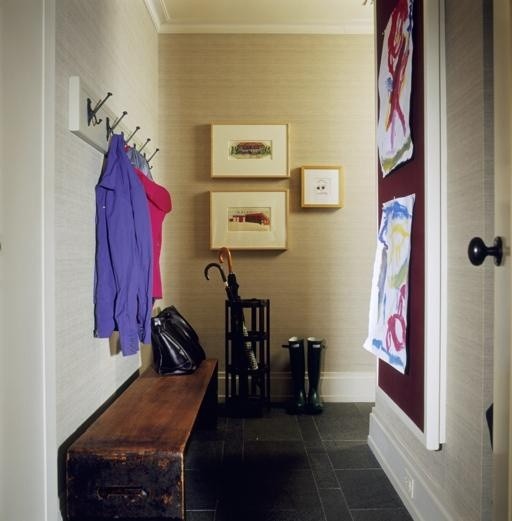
[217,246,250,402]
[203,261,269,399]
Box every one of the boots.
[306,335,325,416]
[280,336,307,416]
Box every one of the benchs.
[62,357,216,521]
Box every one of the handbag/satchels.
[150,303,206,377]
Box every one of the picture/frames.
[209,120,343,251]
